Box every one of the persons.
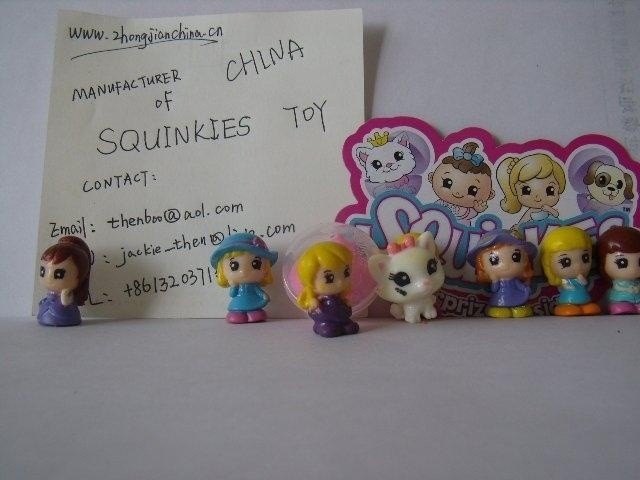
[33,235,91,326]
[295,240,359,337]
[369,229,445,324]
[212,231,277,324]
[598,226,640,315]
[539,225,601,316]
[466,229,539,318]
